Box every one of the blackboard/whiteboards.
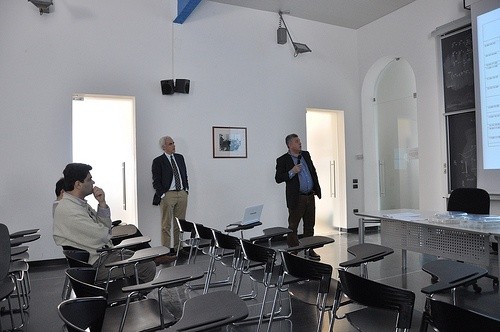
[440,27,475,113]
[446,116,477,193]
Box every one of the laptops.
[231,203,264,226]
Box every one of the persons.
[275,133,321,261]
[152,136,190,249]
[52,178,178,267]
[53,163,156,300]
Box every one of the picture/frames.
[212,126,247,158]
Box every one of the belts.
[300,192,313,195]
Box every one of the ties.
[170,155,181,191]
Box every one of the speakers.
[175,79,190,94]
[161,79,174,95]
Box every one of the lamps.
[293,42,313,58]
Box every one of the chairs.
[0,187,500,332]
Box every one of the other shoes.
[291,252,298,255]
[304,249,320,259]
[174,249,192,258]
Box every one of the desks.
[353,208,500,289]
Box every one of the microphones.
[297,155,302,164]
